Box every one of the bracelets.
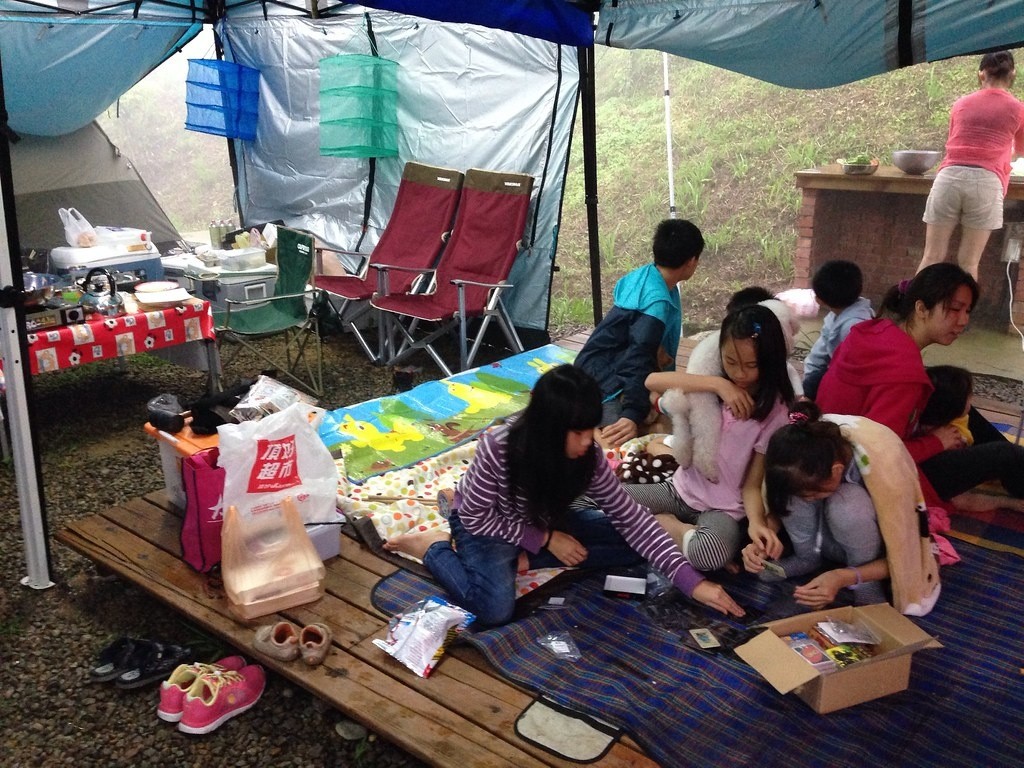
[541,529,553,549]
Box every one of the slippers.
[89,637,199,691]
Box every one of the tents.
[0,1,1023,589]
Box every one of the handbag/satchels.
[181,447,226,574]
[58,207,101,248]
[220,497,326,621]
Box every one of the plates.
[134,280,179,293]
[134,288,192,307]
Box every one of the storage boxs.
[733,603,946,714]
[144,409,346,563]
[51,239,163,285]
[161,247,279,309]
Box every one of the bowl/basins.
[894,149,942,174]
[837,160,878,174]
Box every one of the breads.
[79,232,96,247]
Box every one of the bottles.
[209,219,236,250]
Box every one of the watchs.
[847,566,862,590]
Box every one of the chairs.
[212,162,535,397]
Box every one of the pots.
[23,273,62,307]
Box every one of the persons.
[741,402,940,616]
[382,364,745,629]
[622,263,1024,570]
[572,219,705,449]
[915,51,1024,281]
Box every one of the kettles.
[78,267,126,321]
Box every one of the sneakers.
[157,655,267,735]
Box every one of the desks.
[794,163,1024,336]
[0,293,224,458]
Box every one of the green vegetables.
[846,154,871,165]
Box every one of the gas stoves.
[24,296,85,332]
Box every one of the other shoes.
[300,622,333,665]
[252,622,301,661]
